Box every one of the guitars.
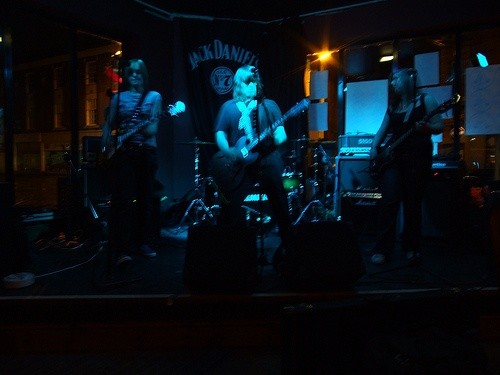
[208,98,312,189]
[370,93,461,179]
[96,100,187,177]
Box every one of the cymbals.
[175,139,215,145]
[290,138,317,143]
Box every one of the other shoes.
[112,252,132,264]
[141,245,157,256]
[371,254,384,266]
[407,252,419,261]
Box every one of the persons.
[100,59,162,266]
[215,65,314,311]
[371,68,445,264]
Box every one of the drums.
[199,176,222,210]
[281,170,303,196]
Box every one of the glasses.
[242,79,256,85]
[128,70,141,76]
